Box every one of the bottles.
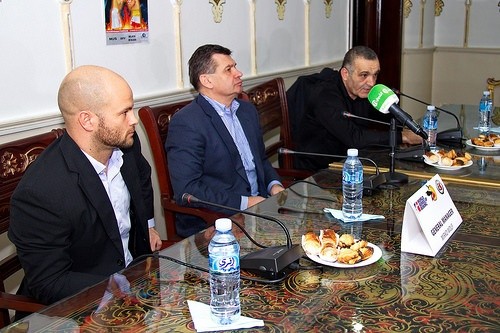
[421,105,438,148]
[341,148,364,219]
[478,91,492,134]
[207,217,242,321]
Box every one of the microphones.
[182,193,302,279]
[368,84,428,140]
[392,86,463,146]
[277,148,386,196]
[344,111,408,130]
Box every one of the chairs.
[0,128,179,328]
[242,77,318,178]
[138,96,261,241]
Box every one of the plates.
[466,139,500,152]
[305,240,383,268]
[423,158,473,170]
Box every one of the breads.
[471,133,500,147]
[302,228,374,265]
[426,150,472,166]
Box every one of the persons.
[7,65,162,322]
[295,46,423,175]
[164,44,286,239]
[194,213,246,258]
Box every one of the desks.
[1,105,500,333]
